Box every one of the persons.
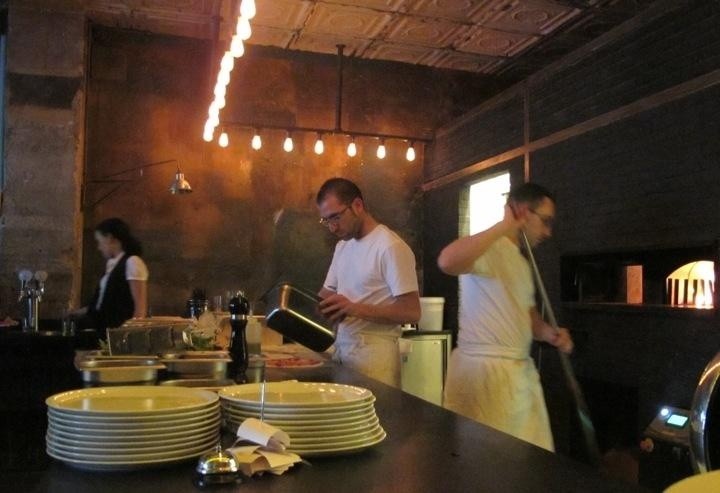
[72,217,150,348]
[438,181,575,452]
[316,177,421,389]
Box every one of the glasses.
[320,207,348,227]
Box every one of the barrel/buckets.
[417,296,445,332]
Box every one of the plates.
[263,361,324,372]
[42,384,223,474]
[217,379,387,455]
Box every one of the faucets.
[16,269,49,331]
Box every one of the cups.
[212,295,224,312]
[61,319,74,336]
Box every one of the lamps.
[82,158,193,212]
[204,0,434,160]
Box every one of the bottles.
[23,287,39,332]
[228,295,250,384]
[245,315,261,356]
[185,299,195,318]
[197,299,209,314]
